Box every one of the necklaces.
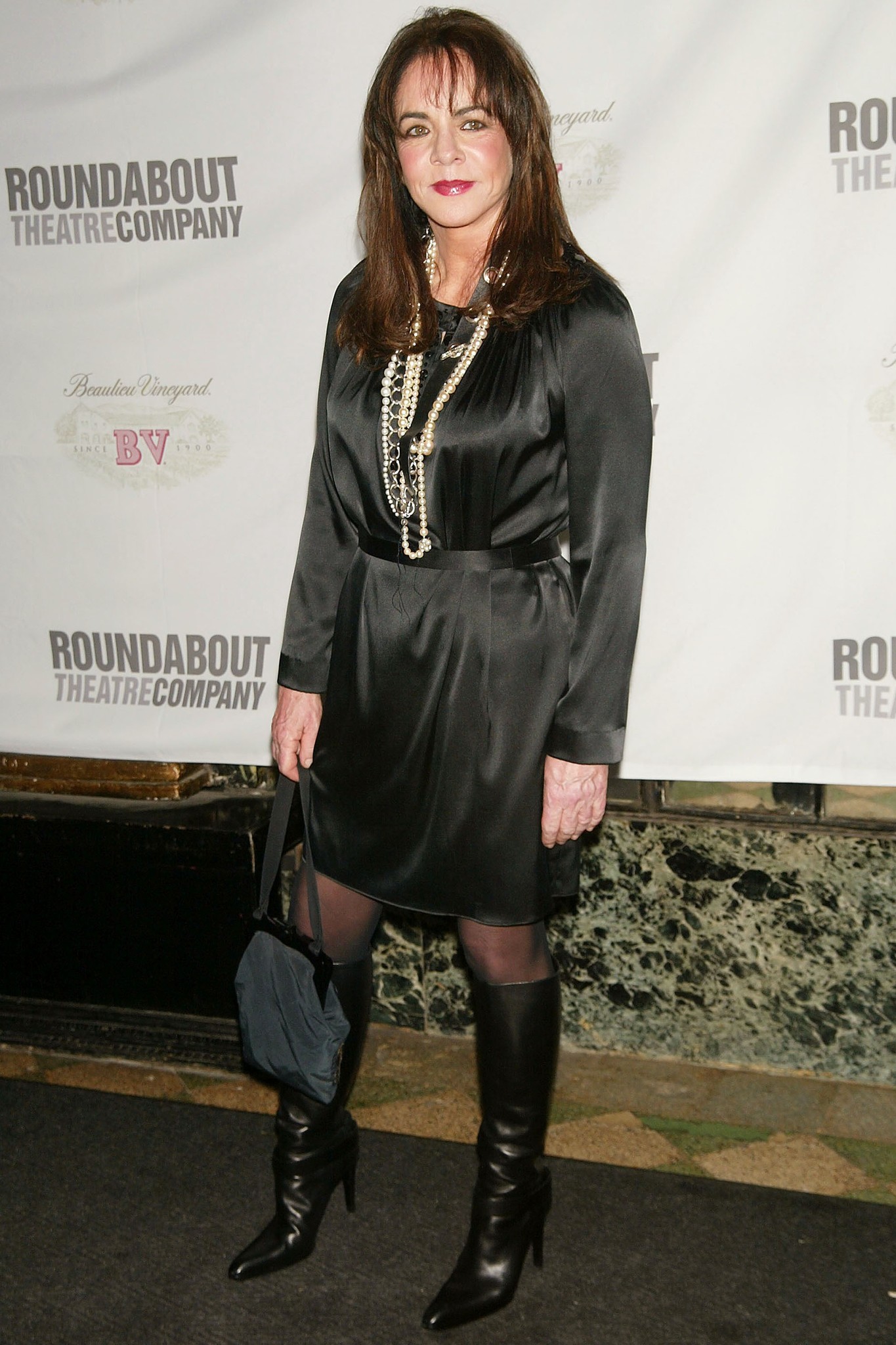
[377,236,511,560]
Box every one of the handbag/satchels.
[231,755,353,1108]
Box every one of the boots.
[420,954,562,1333]
[228,955,374,1281]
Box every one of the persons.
[228,8,653,1328]
